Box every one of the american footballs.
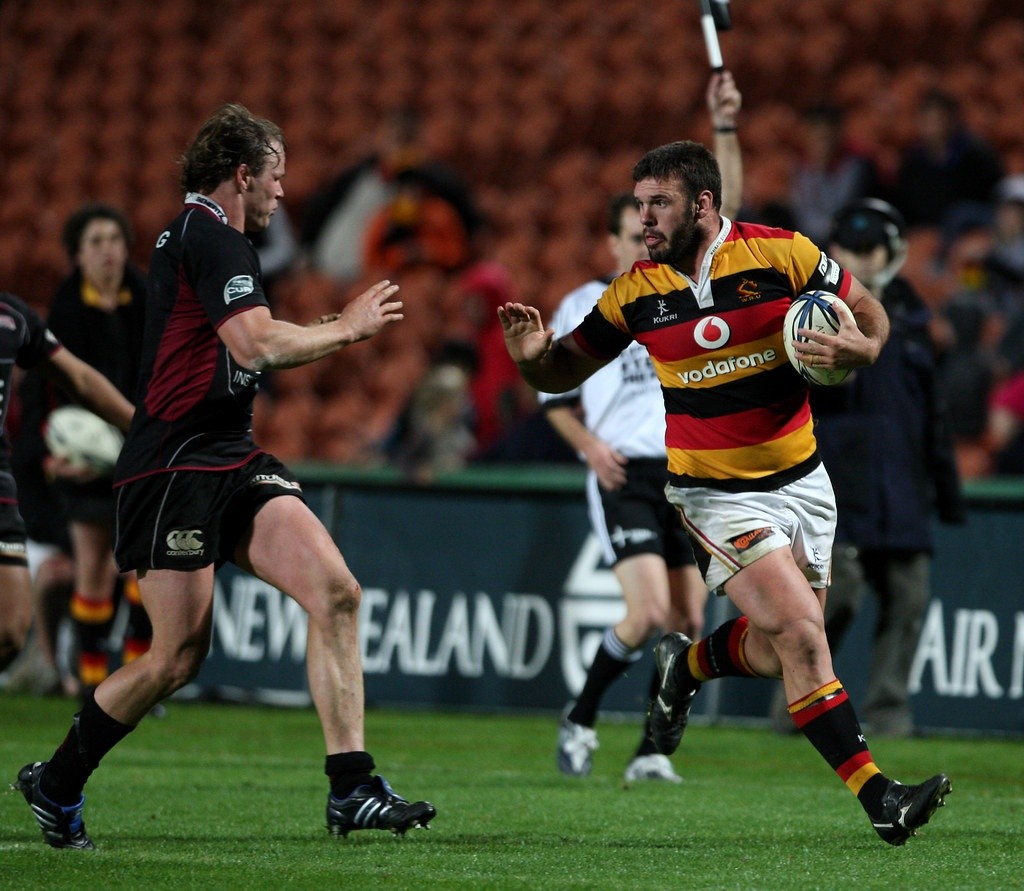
[782,290,859,387]
[44,404,126,477]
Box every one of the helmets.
[832,198,903,264]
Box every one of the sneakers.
[868,773,952,846]
[17,762,95,851]
[326,776,437,842]
[648,632,701,755]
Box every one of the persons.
[0,71,1024,846]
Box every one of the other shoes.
[624,754,683,785]
[557,701,597,778]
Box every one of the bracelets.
[713,127,736,134]
